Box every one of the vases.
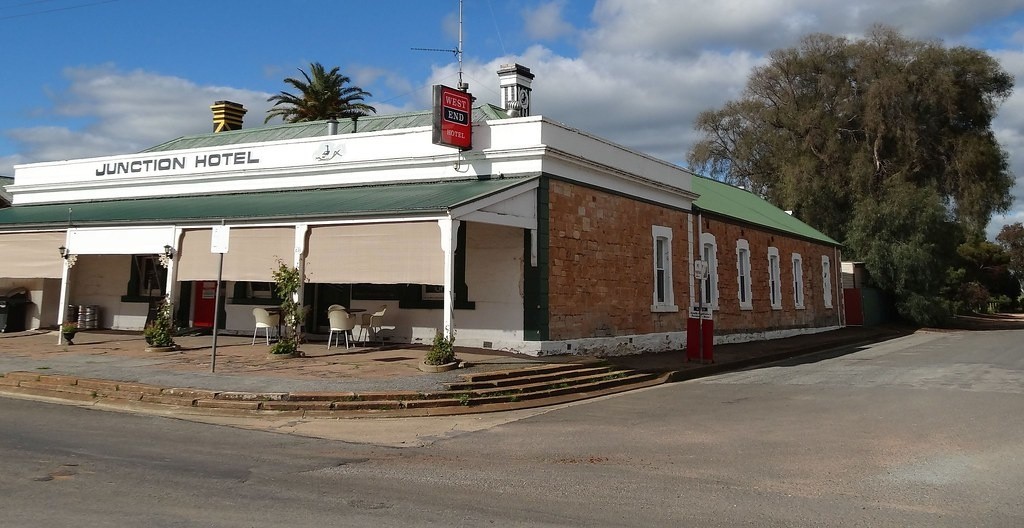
[62,333,75,346]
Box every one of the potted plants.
[143,325,153,346]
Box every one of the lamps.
[58,245,68,260]
[164,244,173,259]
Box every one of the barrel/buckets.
[67,304,100,329]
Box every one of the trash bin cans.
[0,287,29,333]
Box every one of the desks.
[264,308,284,343]
[341,308,368,346]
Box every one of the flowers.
[61,322,77,331]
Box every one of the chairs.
[284,305,310,343]
[328,305,354,344]
[359,305,387,347]
[252,307,279,345]
[326,309,355,350]
